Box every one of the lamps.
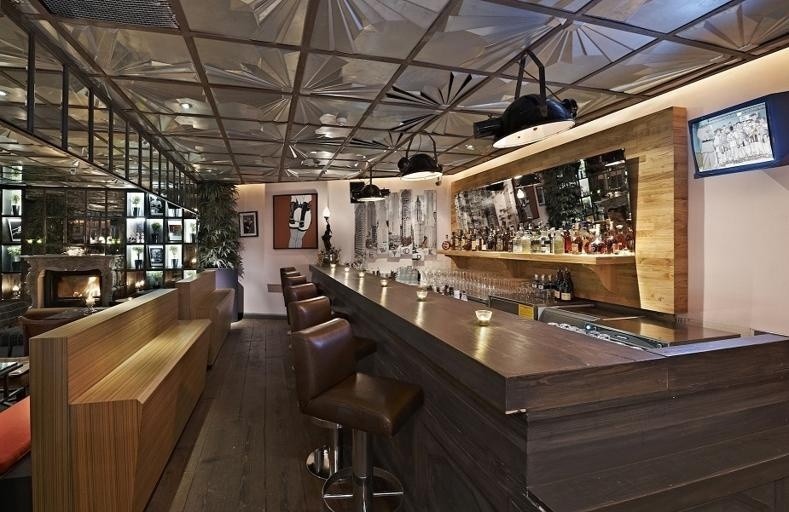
[322,206,333,251]
[516,189,526,206]
[398,131,442,180]
[348,162,390,202]
[474,48,577,151]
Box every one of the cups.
[423,269,533,305]
[474,309,492,326]
[416,290,428,301]
[380,279,388,286]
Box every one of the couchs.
[20,306,112,355]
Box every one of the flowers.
[189,223,196,243]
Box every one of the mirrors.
[452,148,634,251]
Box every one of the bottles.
[441,214,632,254]
[532,266,574,302]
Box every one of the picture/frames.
[535,185,545,206]
[149,247,163,264]
[273,193,318,249]
[7,219,21,242]
[239,211,259,237]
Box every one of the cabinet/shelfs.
[551,168,626,221]
[125,192,200,295]
[2,188,22,300]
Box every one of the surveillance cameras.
[436,180,441,186]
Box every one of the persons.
[288,195,312,248]
[698,111,773,171]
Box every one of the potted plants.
[11,195,20,214]
[169,246,179,268]
[150,223,162,243]
[131,196,140,216]
[5,246,22,272]
[198,183,242,322]
[131,246,143,269]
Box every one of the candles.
[134,280,144,293]
[11,285,19,296]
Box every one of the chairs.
[280,266,423,511]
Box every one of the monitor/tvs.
[688,90,789,179]
